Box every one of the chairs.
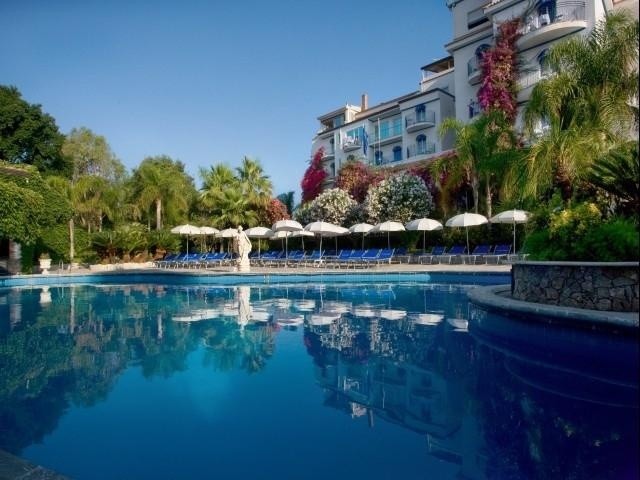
[418,245,446,266]
[465,244,494,265]
[154,246,411,268]
[484,244,513,265]
[441,245,467,265]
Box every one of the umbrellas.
[170,219,351,259]
[349,223,376,252]
[405,217,444,252]
[488,208,533,254]
[445,212,489,255]
[373,220,406,251]
[171,287,469,329]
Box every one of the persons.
[235,226,252,260]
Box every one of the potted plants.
[37,252,52,269]
